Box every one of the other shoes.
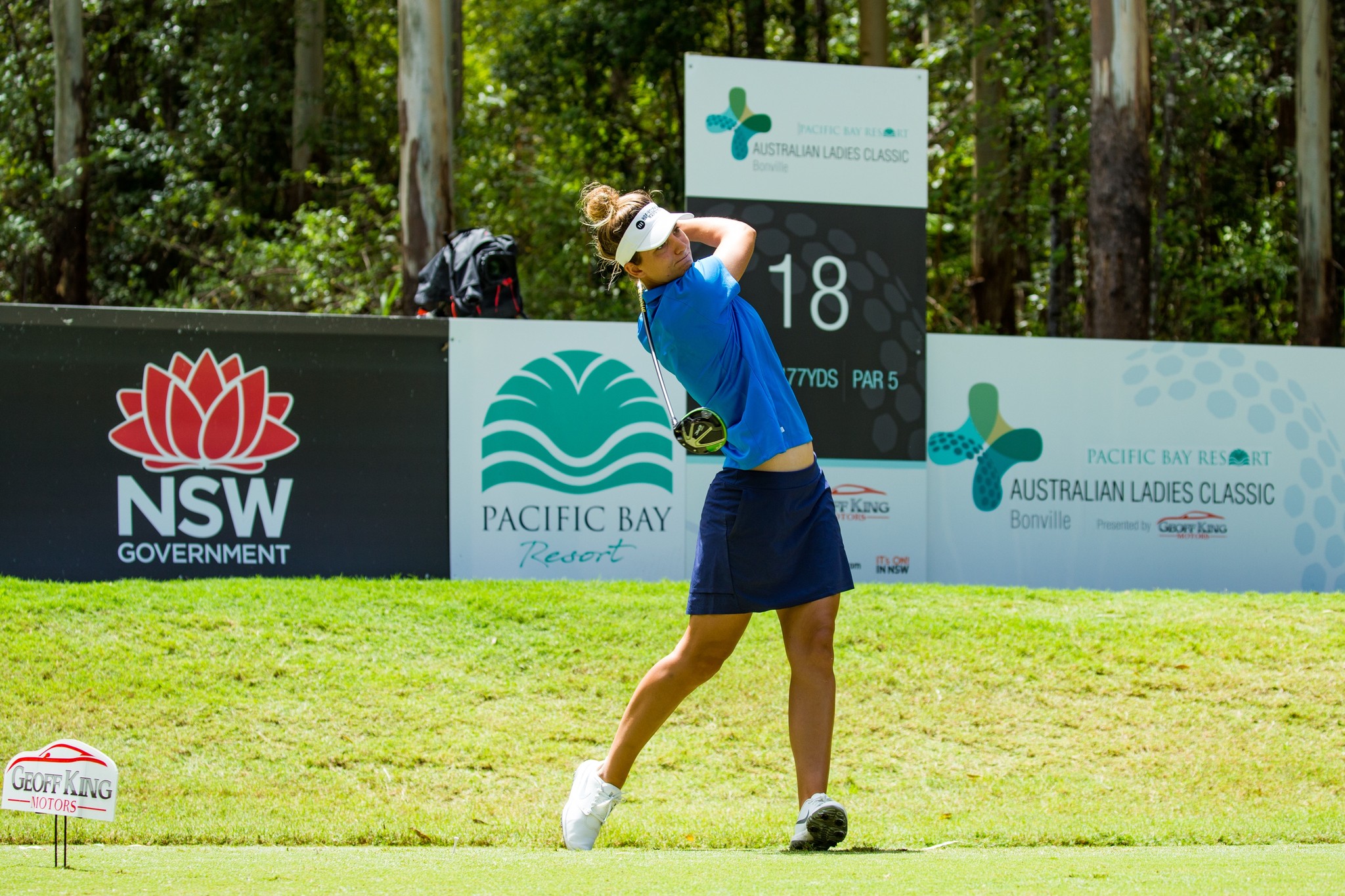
[789,793,848,851]
[560,759,623,850]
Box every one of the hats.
[615,203,694,267]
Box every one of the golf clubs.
[634,280,728,455]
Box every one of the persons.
[558,180,857,853]
